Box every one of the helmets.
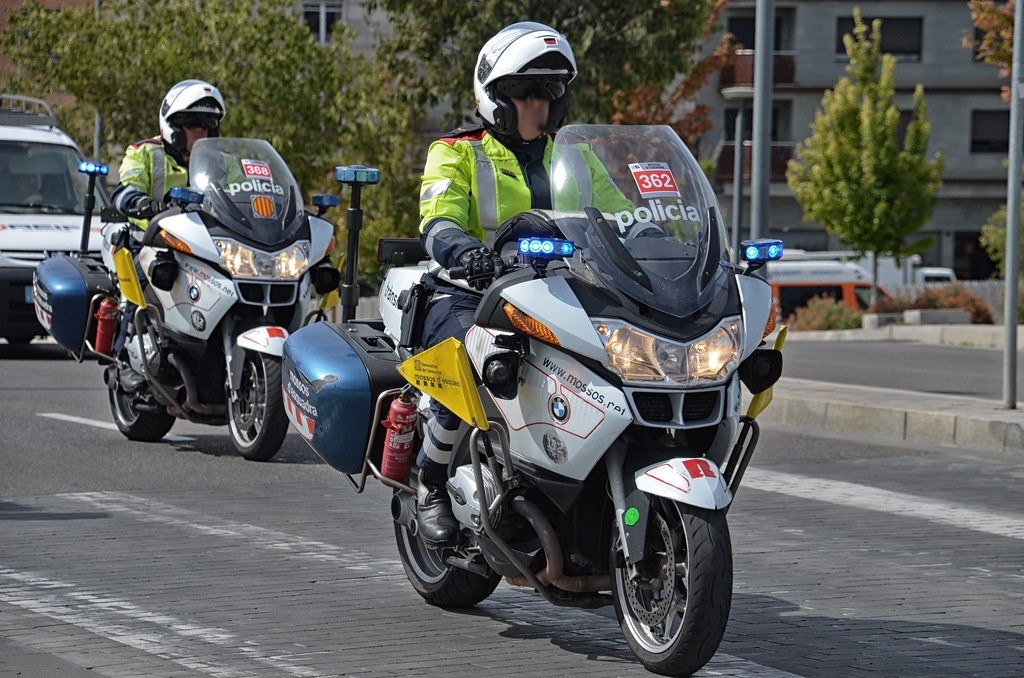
[159,80,227,147]
[473,21,578,136]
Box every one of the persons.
[392,20,704,547]
[101,78,283,392]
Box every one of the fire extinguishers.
[95,285,119,356]
[381,383,418,482]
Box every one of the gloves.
[661,235,696,248]
[458,248,504,291]
[133,194,168,220]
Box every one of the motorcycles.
[30,136,342,465]
[282,122,794,678]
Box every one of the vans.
[752,251,897,335]
[0,93,118,347]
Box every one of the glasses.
[168,111,219,128]
[496,77,566,102]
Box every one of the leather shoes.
[418,468,460,547]
[119,365,147,391]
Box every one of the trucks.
[841,253,960,299]
